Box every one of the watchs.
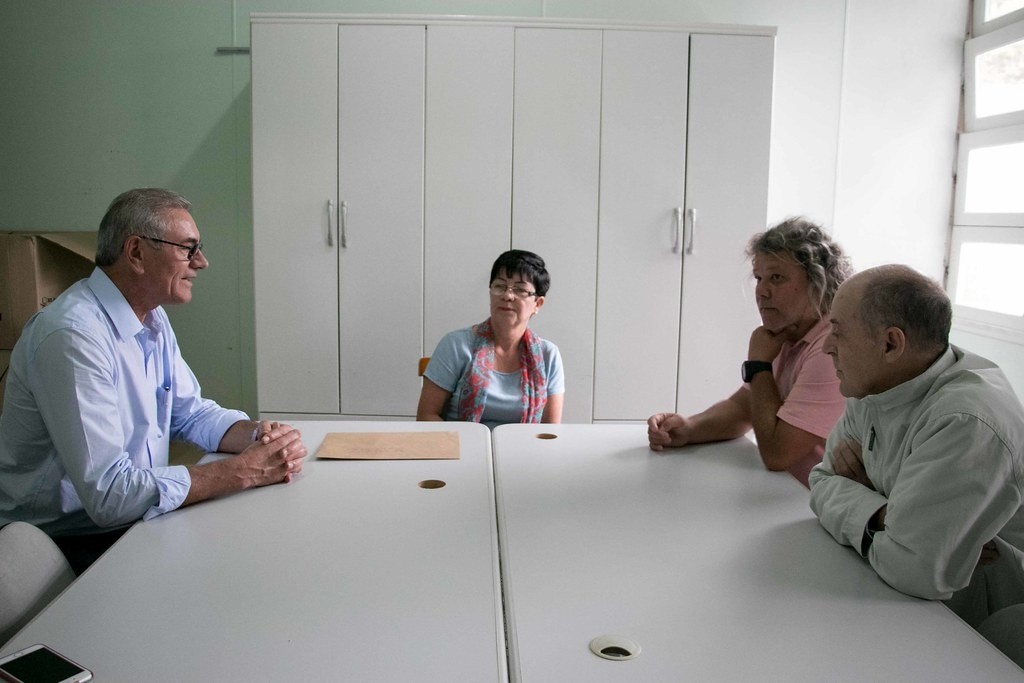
[741,360,773,383]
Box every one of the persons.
[0,187,307,578]
[416,249,564,431]
[809,264,1024,629]
[648,219,847,491]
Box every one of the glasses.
[140,235,204,259]
[490,283,536,298]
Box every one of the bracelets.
[251,426,258,441]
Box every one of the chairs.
[1,518,78,650]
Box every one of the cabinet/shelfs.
[597,21,776,421]
[249,11,427,416]
[422,7,603,425]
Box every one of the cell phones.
[0,643,93,683]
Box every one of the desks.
[0,420,509,683]
[493,423,1024,683]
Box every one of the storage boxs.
[0,231,99,350]
